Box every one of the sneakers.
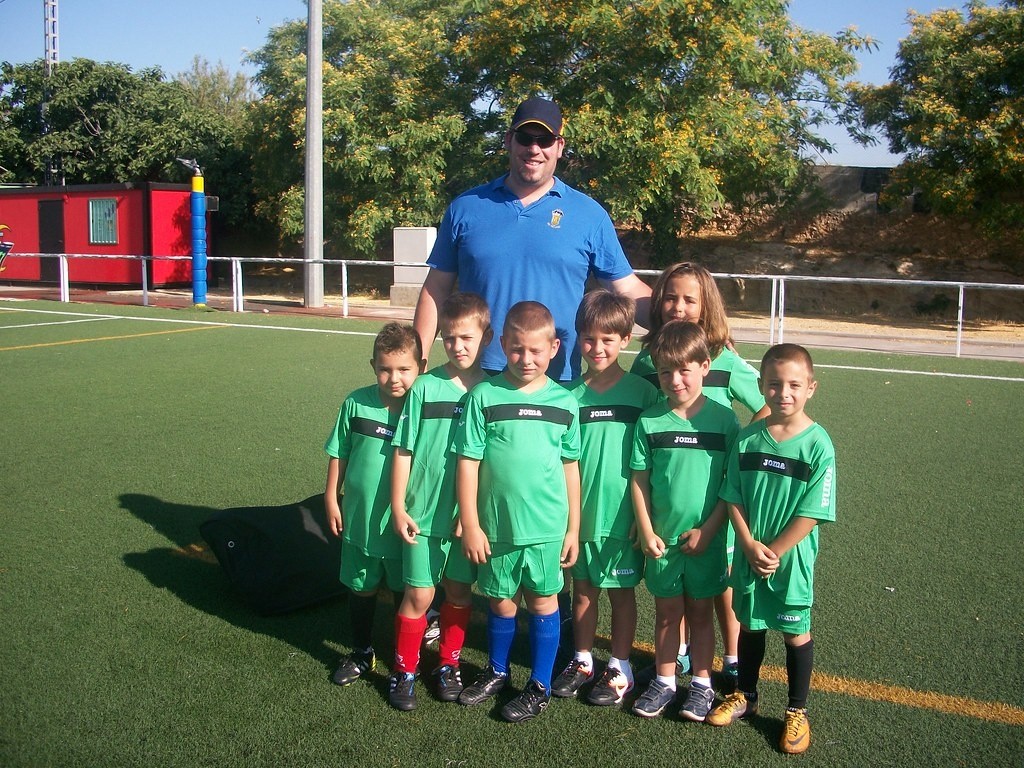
[432,663,463,700]
[390,671,421,711]
[501,677,553,723]
[721,662,741,690]
[459,663,512,707]
[551,659,596,699]
[705,689,757,725]
[680,682,713,720]
[781,707,810,753]
[675,654,690,675]
[588,664,633,707]
[634,677,677,717]
[332,649,376,686]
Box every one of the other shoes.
[423,608,441,643]
[557,606,574,648]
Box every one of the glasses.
[512,130,560,148]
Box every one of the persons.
[389,293,492,712]
[322,324,428,686]
[629,320,740,723]
[450,301,581,722]
[630,261,767,686]
[410,95,736,645]
[551,289,658,705]
[718,345,837,753]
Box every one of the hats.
[513,98,562,137]
